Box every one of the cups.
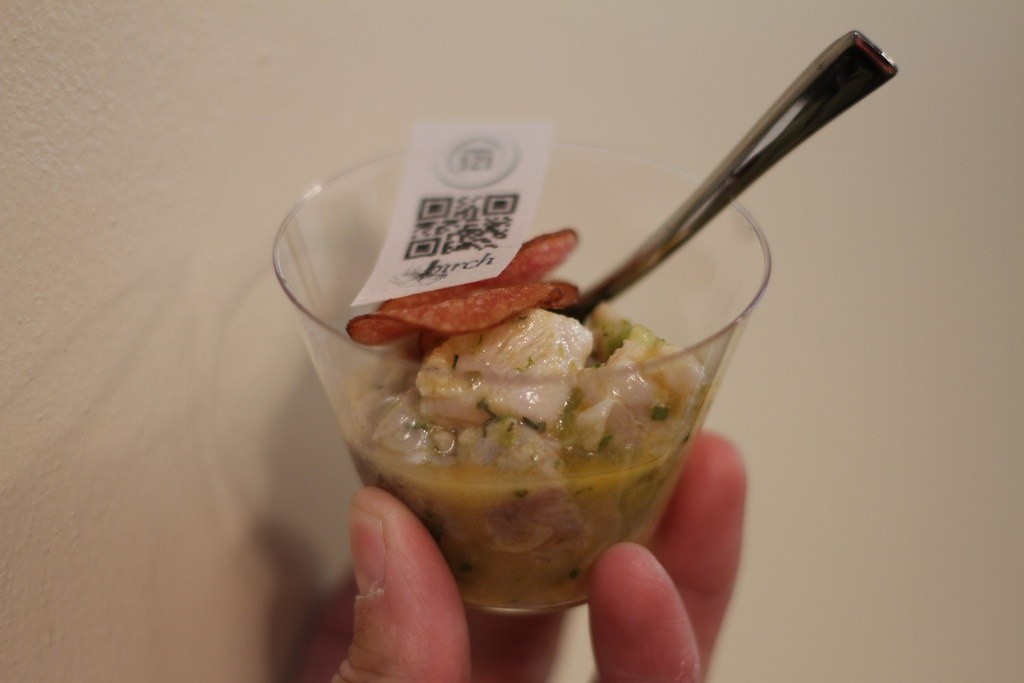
[272,145,772,615]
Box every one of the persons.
[333,432,747,683]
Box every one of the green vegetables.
[378,322,674,581]
